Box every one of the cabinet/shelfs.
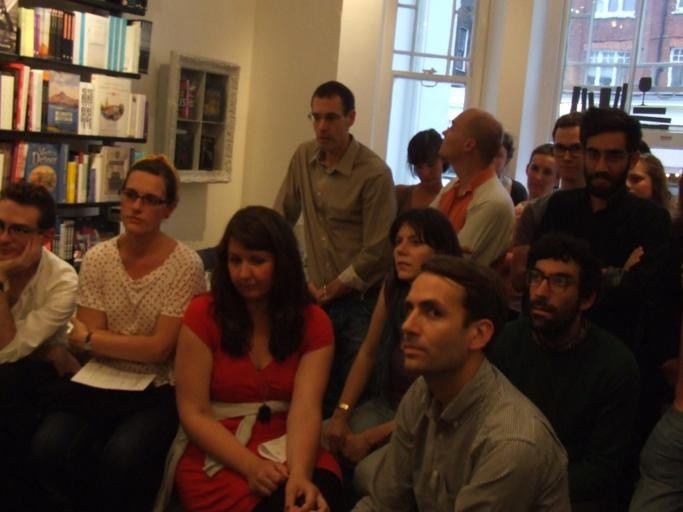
[0,0,152,283]
[152,47,242,187]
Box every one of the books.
[0,0,155,269]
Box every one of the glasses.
[117,187,168,206]
[525,268,580,293]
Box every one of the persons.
[1,155,207,512]
[349,255,571,511]
[273,79,397,360]
[172,206,343,510]
[325,209,457,469]
[394,107,527,276]
[488,104,680,511]
